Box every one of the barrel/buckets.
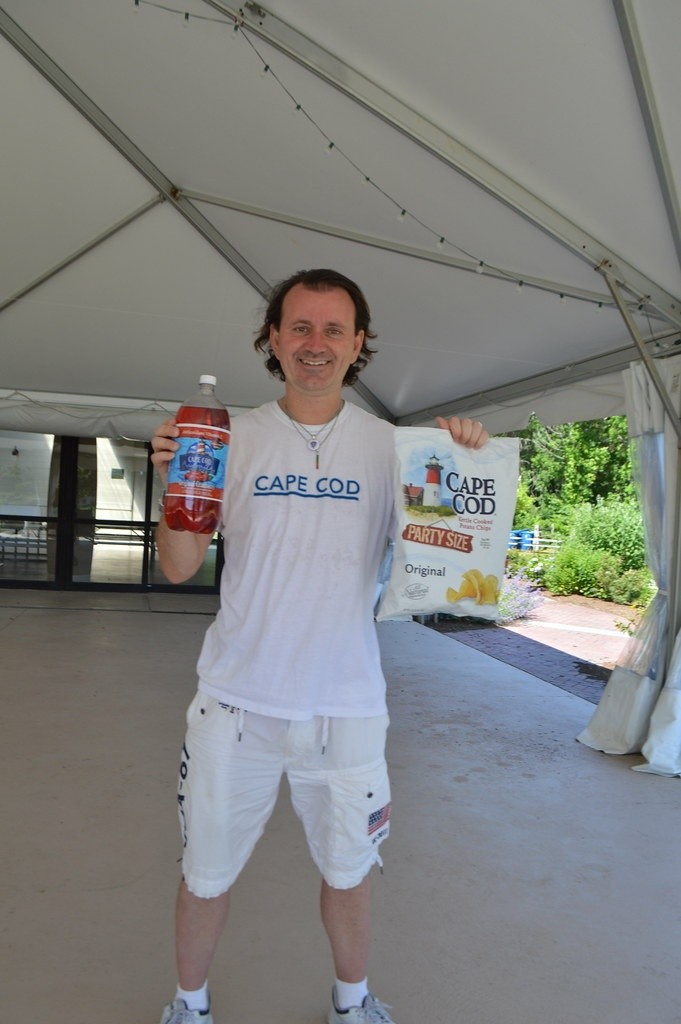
[509,529,534,550]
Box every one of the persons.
[150,268,490,1024]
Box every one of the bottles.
[165,373,230,533]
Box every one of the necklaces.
[282,398,344,451]
[281,396,343,469]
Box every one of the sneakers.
[160,990,213,1024]
[328,985,393,1024]
